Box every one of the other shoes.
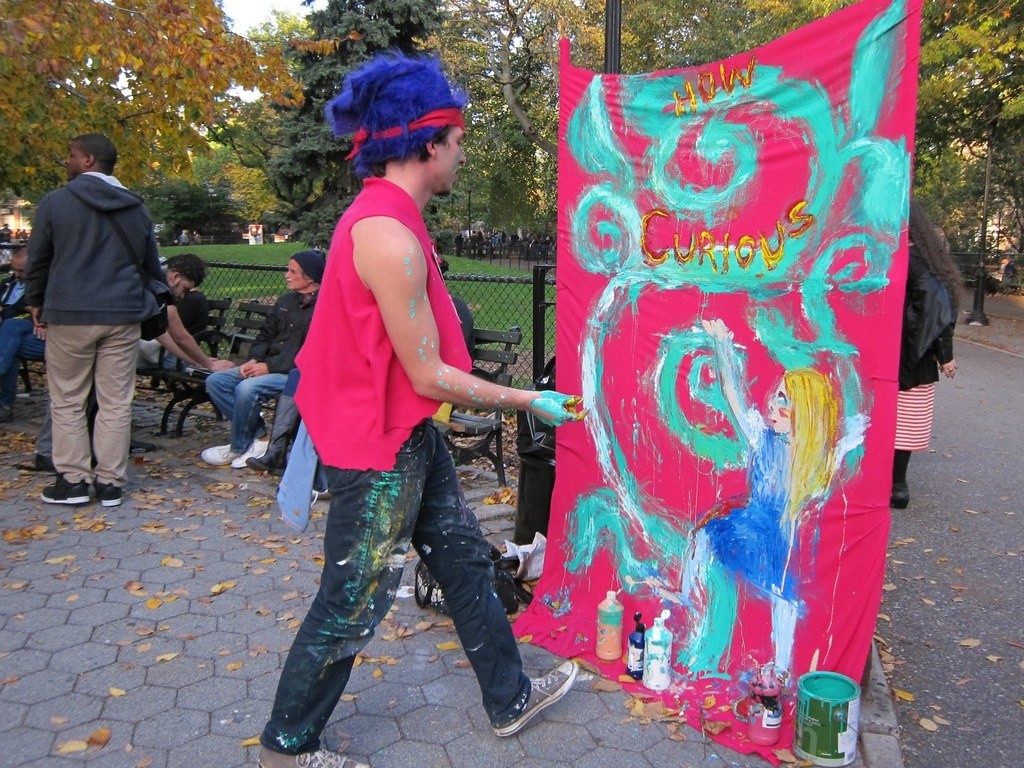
[17,455,55,471]
[310,488,329,506]
[0,406,14,423]
[892,491,910,509]
[91,456,98,469]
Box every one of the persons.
[24,134,168,506]
[175,230,201,245]
[35,254,236,470]
[136,287,208,371]
[889,200,960,511]
[15,229,27,239]
[201,251,327,468]
[0,247,30,322]
[245,257,474,500]
[0,314,45,423]
[0,223,12,243]
[986,256,1015,297]
[454,231,551,261]
[258,60,589,768]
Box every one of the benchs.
[167,300,523,488]
[17,296,232,437]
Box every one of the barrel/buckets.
[792,671,862,766]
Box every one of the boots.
[245,393,302,477]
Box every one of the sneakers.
[200,443,240,466]
[494,661,580,737]
[40,473,90,504]
[230,441,270,467]
[256,746,371,768]
[93,479,122,507]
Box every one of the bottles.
[595,587,624,659]
[748,660,782,745]
[626,611,645,679]
[641,609,673,691]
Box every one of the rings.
[939,367,942,370]
[950,369,953,372]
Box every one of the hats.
[290,250,326,284]
[326,46,463,176]
